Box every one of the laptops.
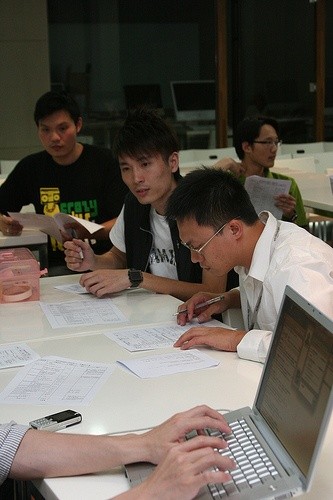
[122,284,333,500]
[123,84,165,116]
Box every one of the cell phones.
[30,410,81,432]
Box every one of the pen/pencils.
[72,228,84,262]
[174,295,224,317]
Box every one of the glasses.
[181,216,240,255]
[247,138,282,146]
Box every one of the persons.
[63,111,240,322]
[161,163,333,365]
[0,405,236,500]
[211,119,310,233]
[0,90,128,277]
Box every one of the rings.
[7,228,9,233]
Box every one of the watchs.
[282,209,298,222]
[128,268,143,288]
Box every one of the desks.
[0,253,333,499]
[177,123,231,150]
[0,178,49,268]
[269,148,332,216]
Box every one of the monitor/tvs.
[169,80,216,121]
